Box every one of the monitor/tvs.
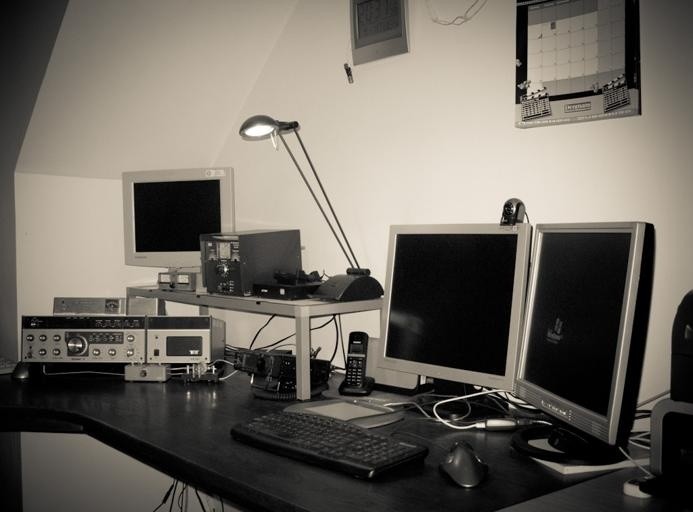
[376,221,531,422]
[515,221,657,466]
[120,166,236,269]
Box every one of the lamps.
[238,115,387,302]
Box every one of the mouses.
[440,439,490,489]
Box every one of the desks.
[1,367,652,512]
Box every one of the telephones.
[339,332,374,395]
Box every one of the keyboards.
[229,409,426,481]
